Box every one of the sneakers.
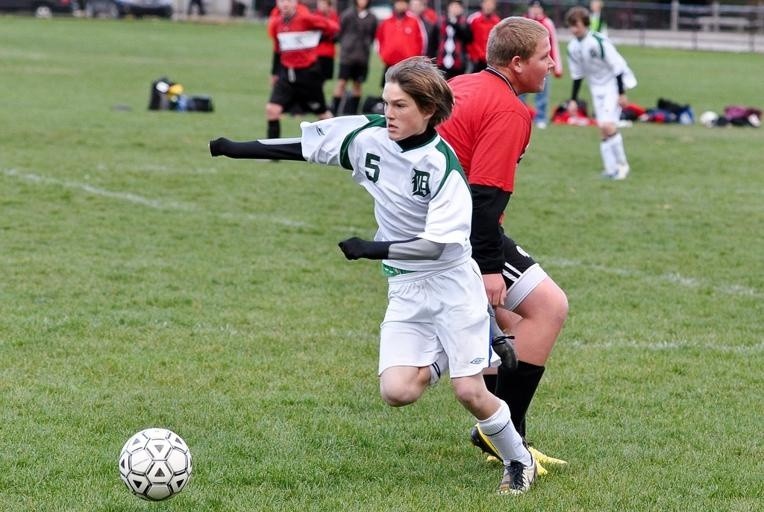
[488,443,567,465]
[488,302,518,370]
[498,449,537,495]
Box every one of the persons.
[210,54,538,493]
[268,0,561,139]
[435,16,569,477]
[564,1,637,180]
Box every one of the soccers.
[118,428,192,500]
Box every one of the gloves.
[338,237,367,260]
